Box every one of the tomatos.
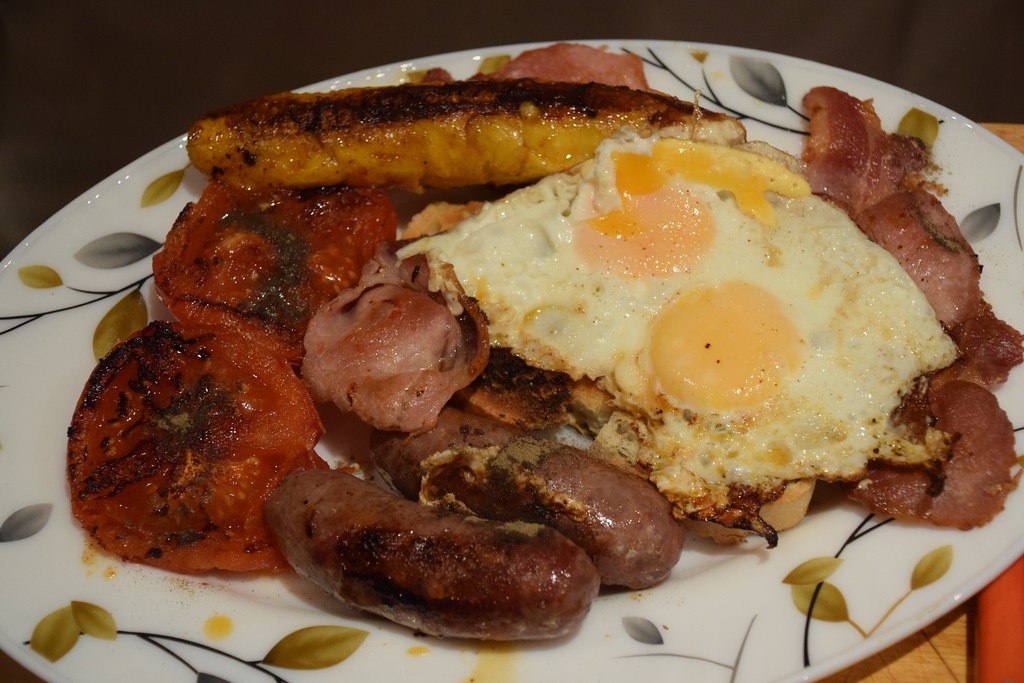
[67,186,397,576]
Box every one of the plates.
[0,37,1024,683]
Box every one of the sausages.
[265,409,681,639]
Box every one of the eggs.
[394,124,959,549]
